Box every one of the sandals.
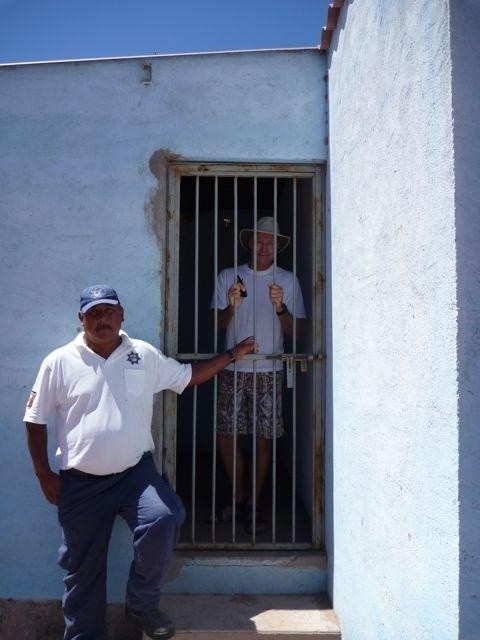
[205,501,267,536]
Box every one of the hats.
[79,284,119,314]
[239,216,291,254]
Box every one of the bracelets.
[275,303,287,315]
[225,349,237,363]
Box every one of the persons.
[210,217,308,534]
[23,285,259,640]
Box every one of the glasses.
[235,274,248,299]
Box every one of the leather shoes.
[124,604,176,640]
